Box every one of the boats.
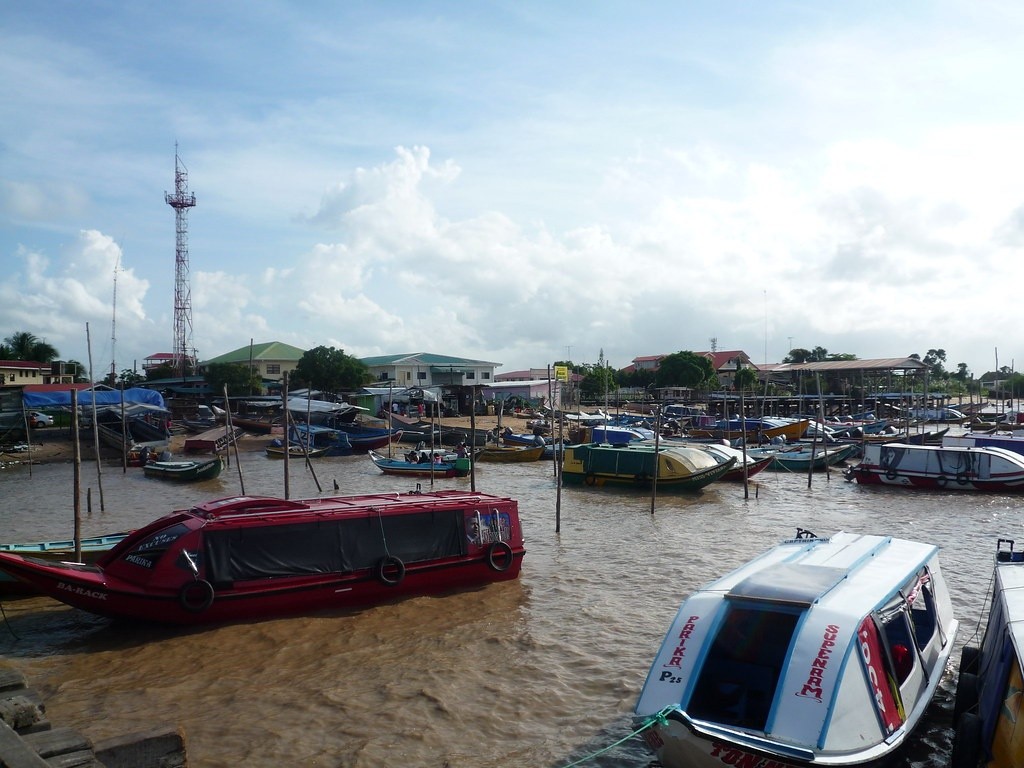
[562,442,737,492]
[264,444,332,458]
[279,396,404,452]
[382,408,493,446]
[513,401,1024,435]
[0,483,528,628]
[98,418,172,466]
[842,432,1024,491]
[739,444,855,471]
[142,455,221,483]
[212,405,272,434]
[479,442,543,462]
[955,539,1024,768]
[500,427,570,458]
[642,438,775,482]
[182,404,216,428]
[0,533,129,583]
[368,449,471,478]
[629,527,959,768]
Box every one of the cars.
[31,411,54,428]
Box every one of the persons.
[672,419,681,434]
[434,453,441,463]
[458,443,469,458]
[399,400,405,412]
[393,401,398,413]
[650,410,654,415]
[409,451,419,464]
[641,418,650,429]
[418,401,424,418]
